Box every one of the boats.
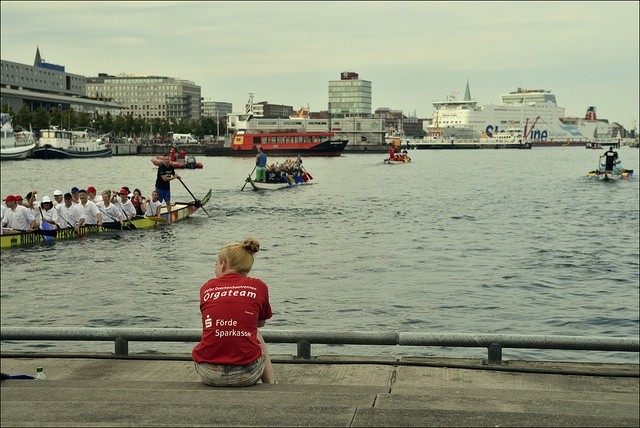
[599,169,622,181]
[249,180,312,190]
[152,156,203,169]
[384,159,412,164]
[34,130,112,159]
[0,144,36,159]
[1,189,212,248]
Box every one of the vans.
[173,134,198,144]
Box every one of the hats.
[53,190,62,196]
[86,187,96,192]
[119,189,127,194]
[42,196,50,203]
[15,196,23,201]
[2,195,16,201]
[71,187,79,192]
[25,192,35,198]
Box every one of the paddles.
[598,156,602,173]
[54,207,84,237]
[178,176,210,217]
[2,228,57,237]
[136,216,167,224]
[240,165,258,191]
[113,191,137,229]
[616,168,633,180]
[148,201,158,224]
[34,192,54,241]
[97,206,131,230]
[276,161,313,185]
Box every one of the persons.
[388,145,409,160]
[192,239,274,386]
[600,147,624,173]
[256,146,302,183]
[0,147,185,234]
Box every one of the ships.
[388,101,624,147]
[199,89,394,154]
[206,131,348,157]
